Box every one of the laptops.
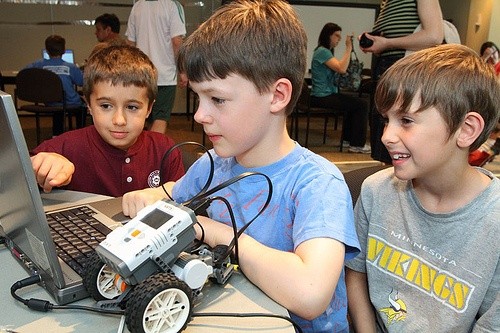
[0,89,133,304]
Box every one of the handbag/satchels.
[333,40,363,91]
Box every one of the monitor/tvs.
[41,48,75,66]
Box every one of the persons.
[176,0,363,333]
[344,42,500,333]
[125,0,187,132]
[30,45,185,218]
[24,35,87,138]
[480,42,500,162]
[89,14,130,58]
[311,23,372,153]
[358,0,445,163]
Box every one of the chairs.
[290,79,339,149]
[14,67,84,146]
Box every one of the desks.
[0,189,297,333]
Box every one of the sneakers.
[348,145,371,153]
[343,141,350,147]
